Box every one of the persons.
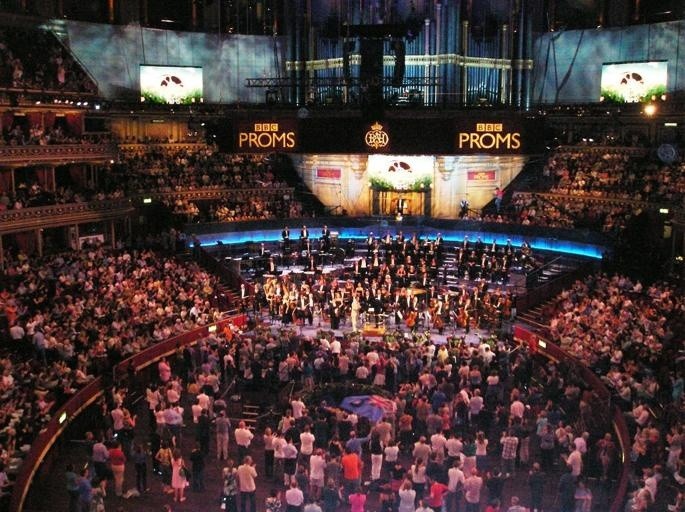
[1,29,684,511]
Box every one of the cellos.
[405,287,512,329]
